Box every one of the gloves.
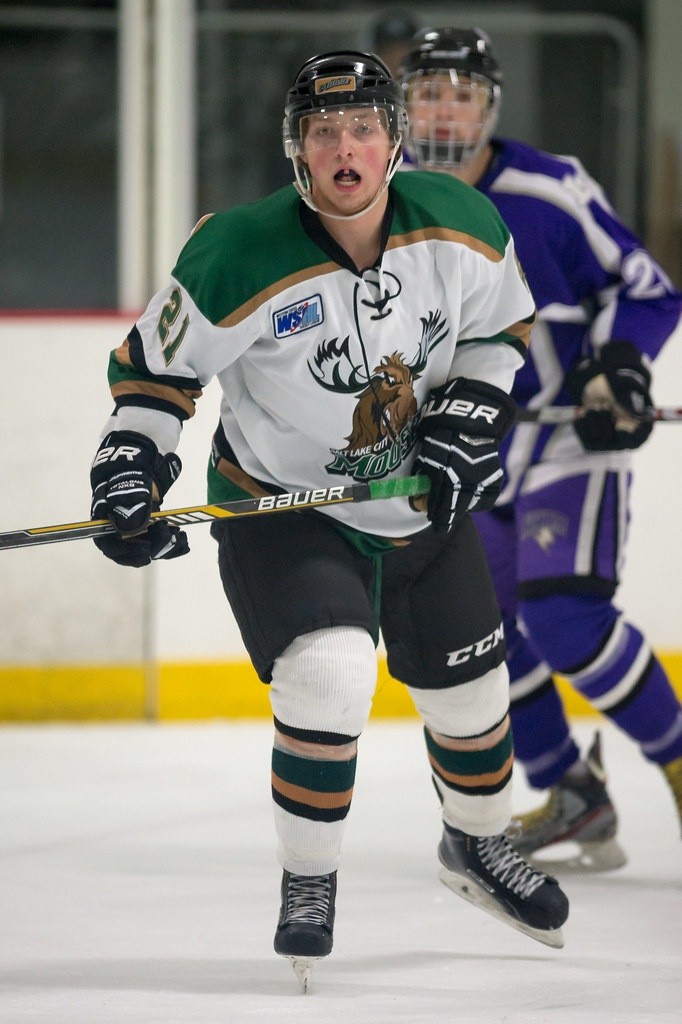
[408,376,518,538]
[90,430,190,567]
[571,339,654,453]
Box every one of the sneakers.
[662,756,682,816]
[503,729,628,878]
[274,868,338,993]
[432,776,569,949]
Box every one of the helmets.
[283,50,407,163]
[395,26,504,170]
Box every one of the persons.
[390,21,681,873]
[89,48,573,996]
